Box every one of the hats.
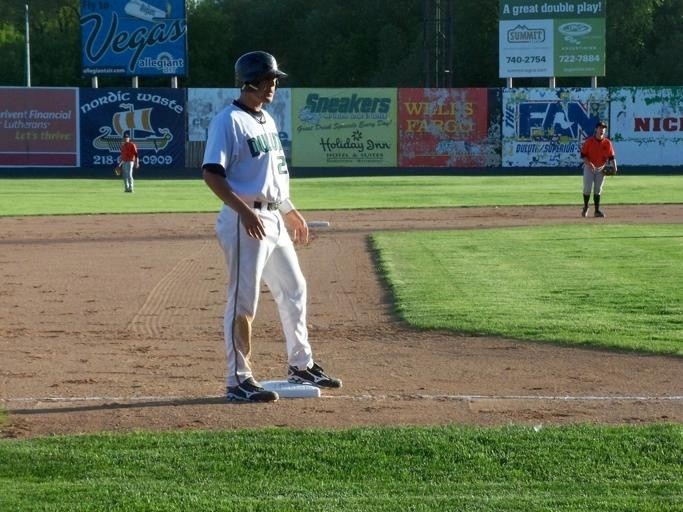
[596,121,608,129]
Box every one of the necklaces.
[236,99,263,125]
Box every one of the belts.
[222,200,281,211]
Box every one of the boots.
[581,193,591,218]
[593,194,605,217]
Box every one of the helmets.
[233,50,288,89]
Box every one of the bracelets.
[277,199,294,216]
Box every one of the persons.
[118,132,139,193]
[580,121,618,218]
[201,49,343,403]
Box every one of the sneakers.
[286,361,343,390]
[225,376,280,404]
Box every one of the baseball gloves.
[114,166,124,175]
[603,166,616,176]
